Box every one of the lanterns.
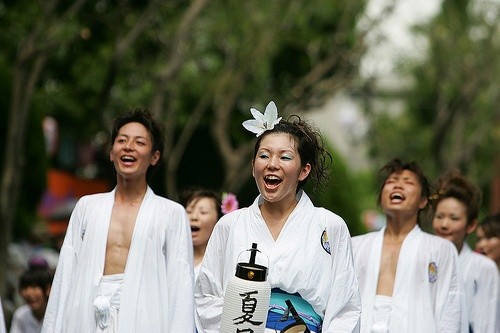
[219,262,271,333]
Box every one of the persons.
[193,101,362,333]
[9,255,54,333]
[185,188,237,284]
[41,105,195,333]
[429,175,500,333]
[351,154,462,333]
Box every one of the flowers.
[221,192,239,214]
[242,100,283,137]
